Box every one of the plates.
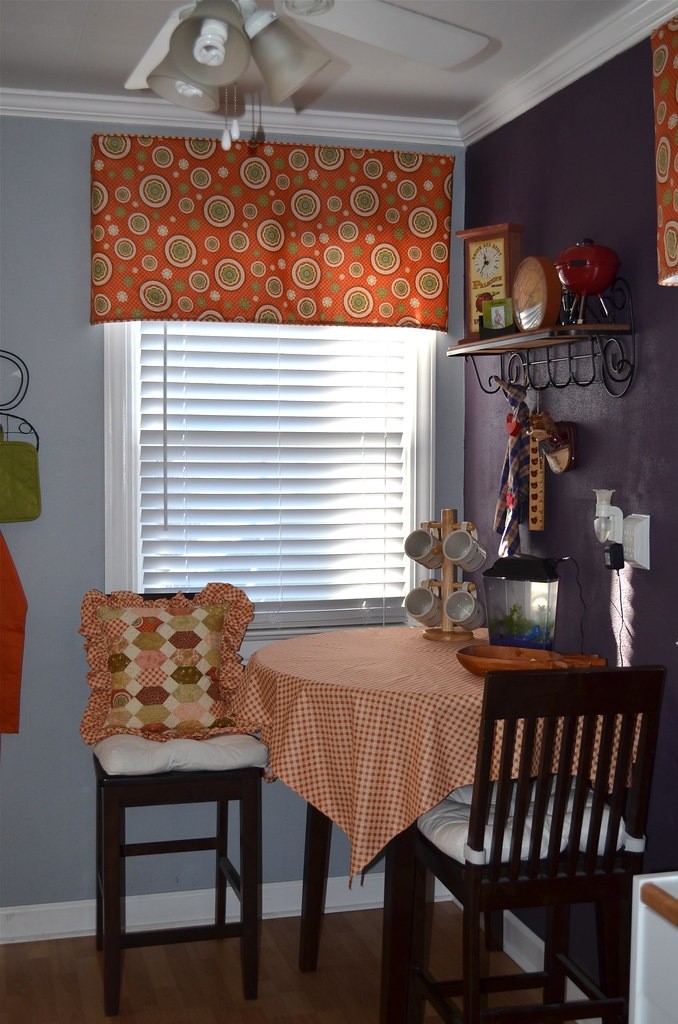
[457,645,608,674]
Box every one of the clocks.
[512,255,562,333]
[454,222,528,345]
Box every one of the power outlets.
[623,513,650,570]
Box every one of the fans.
[118,0,491,91]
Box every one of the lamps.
[146,0,332,151]
[592,487,623,544]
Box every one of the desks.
[230,625,643,1024]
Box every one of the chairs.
[413,663,667,1024]
[79,592,269,1017]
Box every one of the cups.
[404,521,443,569]
[443,521,486,572]
[405,579,442,627]
[444,582,486,628]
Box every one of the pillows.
[78,582,263,746]
